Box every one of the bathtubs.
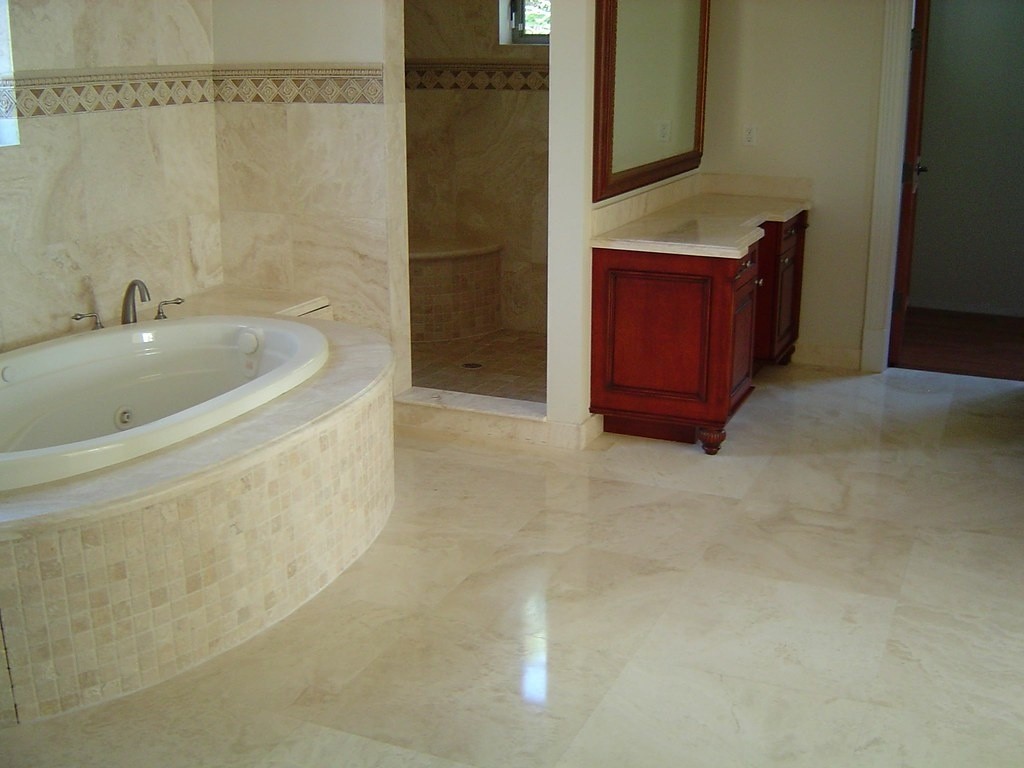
[0,316,395,725]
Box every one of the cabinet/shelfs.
[588,192,814,457]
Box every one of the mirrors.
[592,0,711,203]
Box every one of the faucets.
[121,280,151,324]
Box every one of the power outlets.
[742,124,758,147]
[655,119,672,142]
